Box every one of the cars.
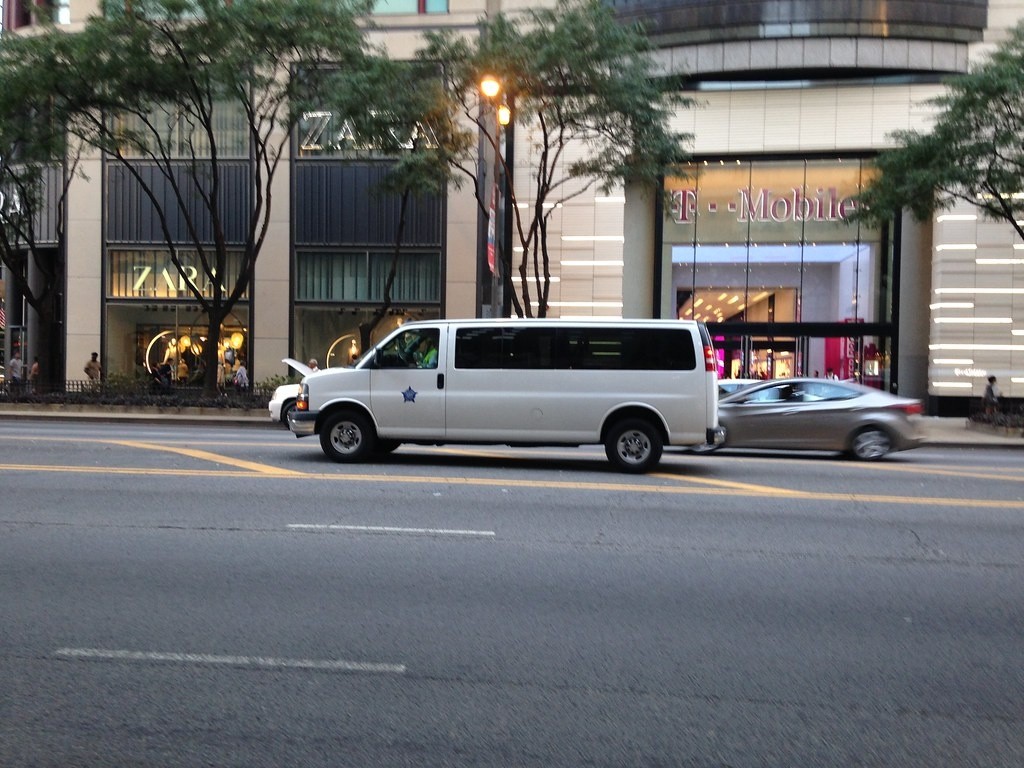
[268,358,313,428]
[714,377,823,403]
[686,377,924,463]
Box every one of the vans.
[288,318,726,475]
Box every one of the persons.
[813,371,819,378]
[236,360,247,387]
[152,358,188,394]
[825,368,839,381]
[308,359,319,371]
[84,352,101,393]
[216,361,227,397]
[409,336,438,369]
[9,352,39,393]
[984,376,1001,414]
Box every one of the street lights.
[478,73,513,317]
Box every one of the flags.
[0,297,6,327]
